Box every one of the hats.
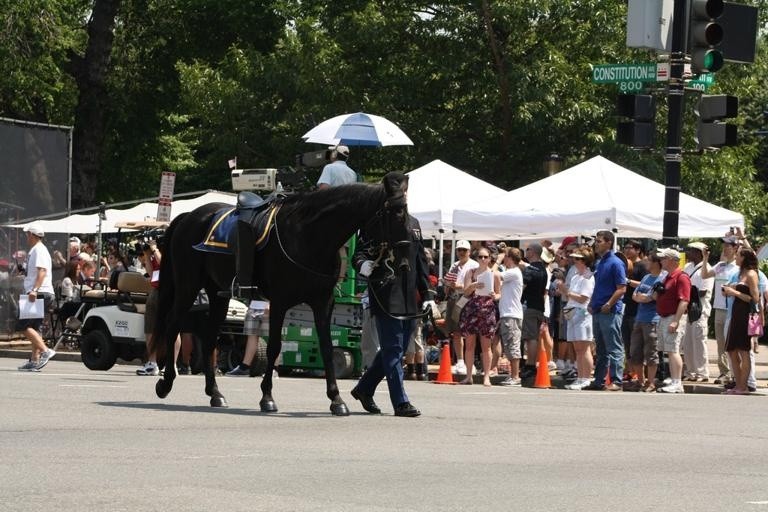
[718,236,739,247]
[540,247,555,263]
[687,242,708,251]
[656,247,680,261]
[328,145,349,157]
[569,253,584,258]
[614,252,633,277]
[456,240,471,250]
[23,225,45,237]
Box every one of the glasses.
[477,255,489,259]
[457,248,467,251]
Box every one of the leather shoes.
[351,386,381,413]
[394,402,421,417]
[407,372,426,380]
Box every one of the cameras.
[654,282,665,293]
[722,285,726,291]
[730,226,734,235]
[707,244,713,251]
[497,242,505,248]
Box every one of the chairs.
[47,281,81,348]
[79,270,152,314]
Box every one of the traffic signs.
[686,72,715,92]
[591,62,657,93]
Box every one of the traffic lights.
[699,94,738,147]
[690,0,724,74]
[616,93,656,148]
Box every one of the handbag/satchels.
[747,312,764,337]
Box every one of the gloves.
[358,260,379,277]
[421,299,442,319]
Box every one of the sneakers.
[137,361,192,376]
[262,368,279,378]
[451,364,536,387]
[225,364,250,377]
[682,376,709,382]
[18,348,56,371]
[548,361,578,381]
[714,374,755,394]
[563,377,684,393]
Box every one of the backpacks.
[687,285,702,322]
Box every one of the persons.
[225,300,279,378]
[0,250,27,289]
[136,239,181,377]
[51,237,149,312]
[404,231,768,395]
[18,223,56,371]
[360,287,381,379]
[181,288,223,377]
[351,171,442,417]
[317,145,356,282]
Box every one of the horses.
[147,176,415,417]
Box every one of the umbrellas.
[301,111,414,185]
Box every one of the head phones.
[330,144,340,162]
